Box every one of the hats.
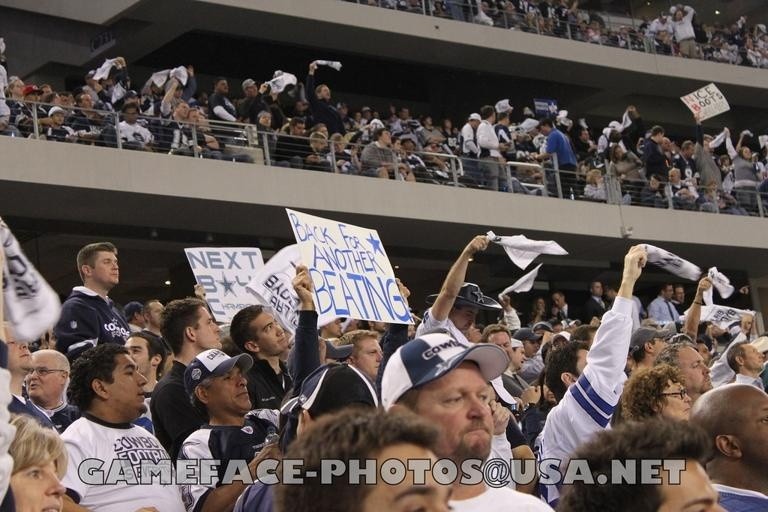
[281,337,379,420]
[183,349,255,396]
[425,282,503,311]
[511,319,582,348]
[629,325,670,355]
[381,333,511,414]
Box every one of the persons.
[1,0,768,512]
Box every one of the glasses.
[656,388,688,399]
[28,366,64,375]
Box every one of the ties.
[665,301,674,320]
